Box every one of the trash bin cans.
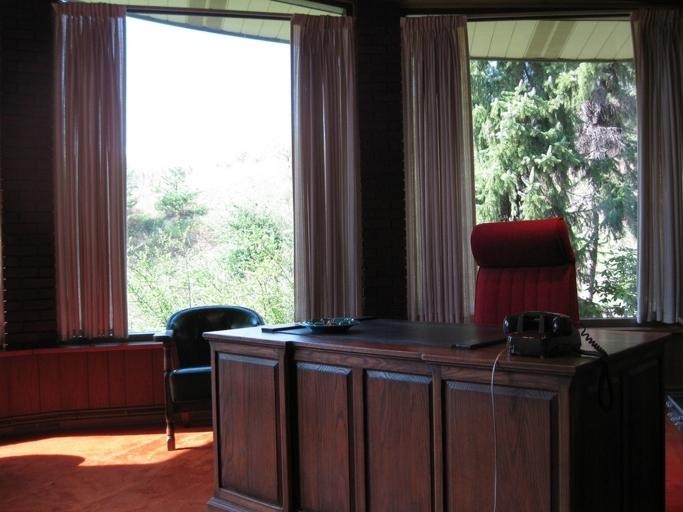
[664,383,682,510]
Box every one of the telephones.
[502,309,581,356]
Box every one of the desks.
[199,316,671,512]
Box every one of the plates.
[298,317,360,334]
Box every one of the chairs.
[150,304,268,452]
[469,218,583,330]
